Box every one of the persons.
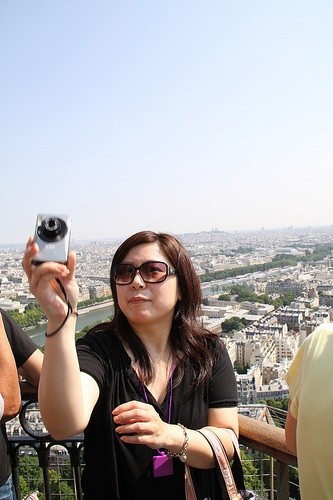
[284,306,333,500]
[0,307,44,500]
[0,310,21,422]
[23,230,240,499]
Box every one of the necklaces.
[133,347,173,477]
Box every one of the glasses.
[111,260,180,285]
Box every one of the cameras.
[31,213,71,265]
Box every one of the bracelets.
[165,423,188,462]
